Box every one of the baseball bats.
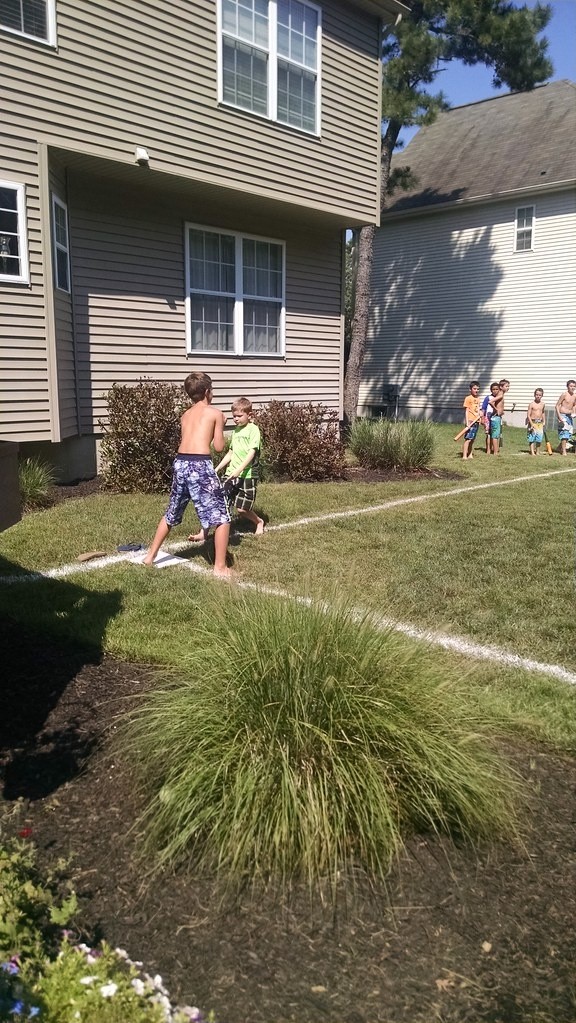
[544,431,552,455]
[454,416,481,441]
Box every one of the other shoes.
[78,551,107,560]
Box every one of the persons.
[141,371,247,577]
[555,380,576,456]
[482,379,510,456]
[527,388,545,456]
[188,397,264,541]
[462,381,484,460]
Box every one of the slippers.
[117,541,146,551]
